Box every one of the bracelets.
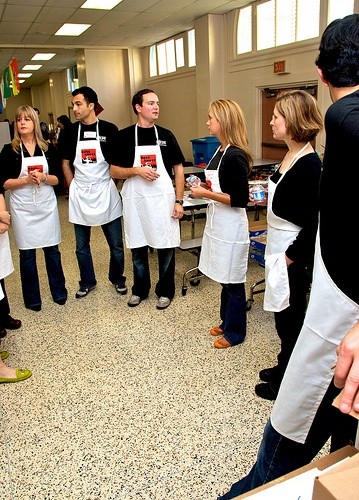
[43,173,48,182]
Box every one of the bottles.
[252,180,266,205]
[188,174,201,187]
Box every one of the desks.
[179,181,268,240]
[172,159,280,175]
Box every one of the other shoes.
[76,284,95,298]
[115,283,127,295]
[156,296,171,309]
[128,294,148,307]
[0,368,32,383]
[210,326,224,336]
[0,317,21,338]
[30,304,41,311]
[255,382,279,401]
[214,336,231,348]
[259,367,276,383]
[57,298,66,305]
[0,351,9,360]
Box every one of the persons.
[4,119,14,141]
[60,86,128,299]
[186,98,254,349]
[33,108,40,116]
[255,90,324,401]
[0,177,21,338]
[0,351,32,384]
[0,105,68,311]
[109,89,185,310]
[218,13,359,500]
[57,115,71,144]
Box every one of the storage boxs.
[248,230,267,268]
[313,452,359,500]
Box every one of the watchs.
[175,200,183,205]
